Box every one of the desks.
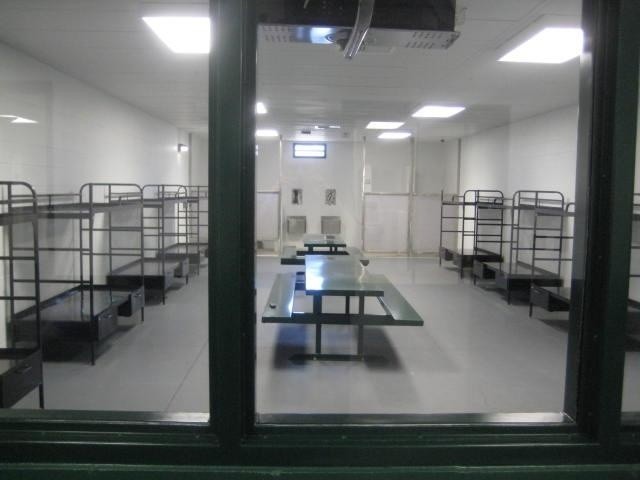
[303,232,347,265]
[290,254,385,362]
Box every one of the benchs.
[345,247,369,266]
[371,272,427,326]
[280,245,298,265]
[260,271,299,325]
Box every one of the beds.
[437,189,640,351]
